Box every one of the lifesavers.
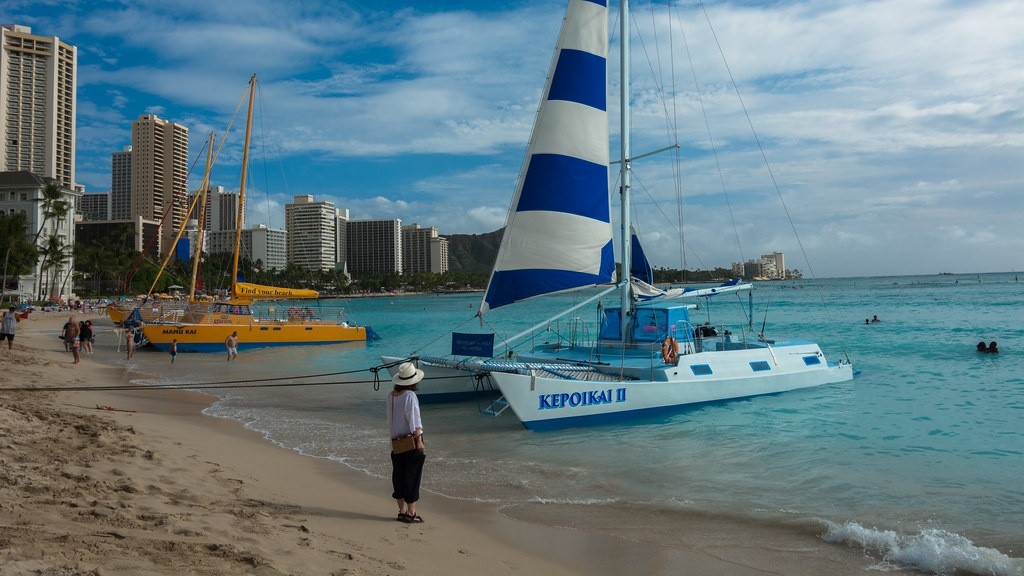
[663,337,679,363]
[287,307,299,318]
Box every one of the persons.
[62,317,95,364]
[288,308,315,322]
[977,342,998,354]
[224,331,238,361]
[695,322,717,337]
[866,319,869,324]
[0,307,18,349]
[169,339,177,364]
[125,327,135,359]
[872,315,880,322]
[386,362,426,524]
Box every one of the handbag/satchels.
[392,434,417,454]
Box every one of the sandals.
[397,510,408,521]
[404,511,424,523]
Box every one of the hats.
[392,362,425,386]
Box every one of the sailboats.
[104,72,375,354]
[382,0,856,431]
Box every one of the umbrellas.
[137,285,219,299]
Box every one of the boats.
[445,284,486,293]
[432,286,446,294]
[752,275,771,281]
